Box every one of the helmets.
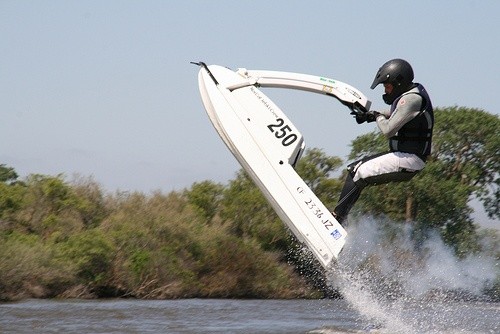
[370,58,414,105]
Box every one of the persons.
[332,59,434,225]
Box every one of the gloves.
[366,110,381,123]
[356,112,367,124]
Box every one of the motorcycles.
[190,61,374,268]
